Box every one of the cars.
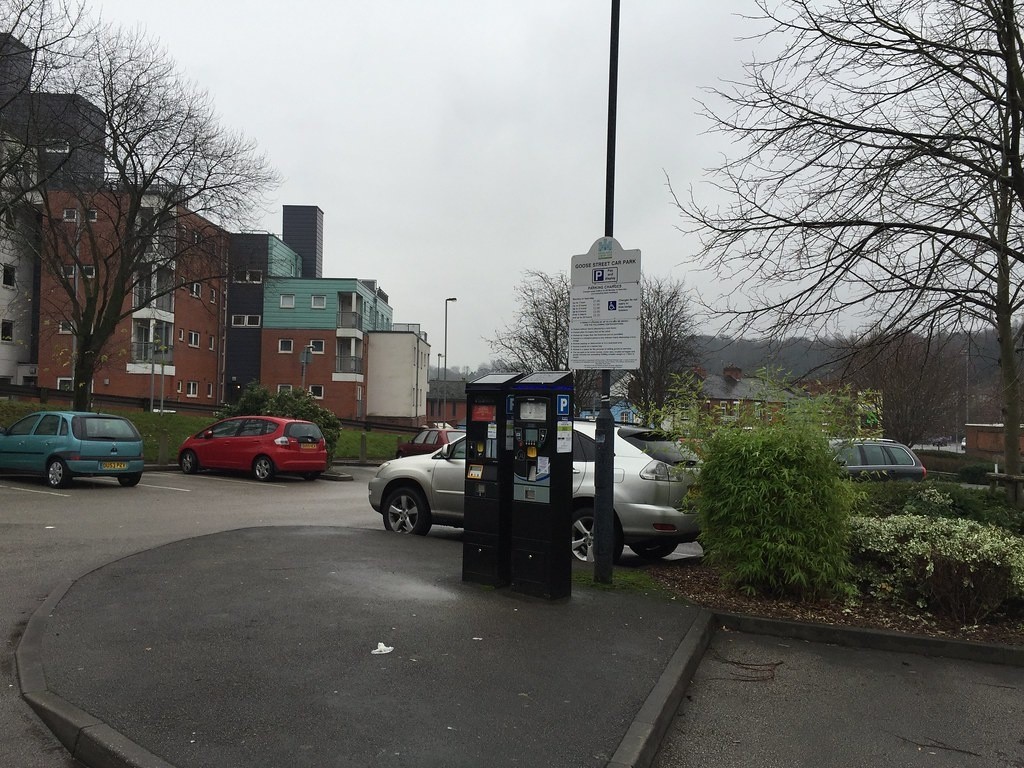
[422,423,455,430]
[177,414,328,481]
[679,438,712,463]
[0,409,146,488]
[394,428,465,460]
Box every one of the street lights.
[301,344,315,394]
[436,354,445,429]
[442,298,458,428]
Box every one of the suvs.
[821,437,927,485]
[366,418,714,566]
